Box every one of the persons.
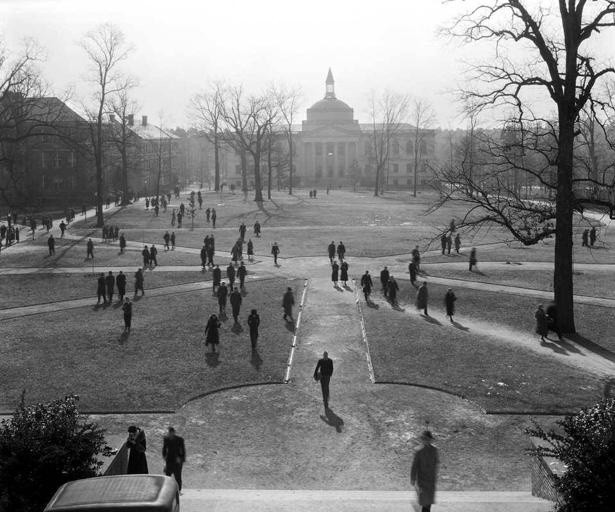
[283,287,294,321]
[247,309,260,346]
[231,238,254,263]
[535,302,549,342]
[200,234,215,268]
[0,197,126,259]
[163,231,176,250]
[213,261,247,322]
[583,228,589,245]
[122,297,132,331]
[411,430,440,511]
[327,232,478,321]
[313,352,333,408]
[309,190,313,198]
[239,223,246,240]
[450,218,456,233]
[313,188,317,198]
[97,268,144,303]
[144,186,216,227]
[590,228,597,246]
[162,427,185,491]
[125,425,148,474]
[205,314,221,347]
[254,221,261,236]
[142,245,157,267]
[546,300,562,340]
[271,242,280,264]
